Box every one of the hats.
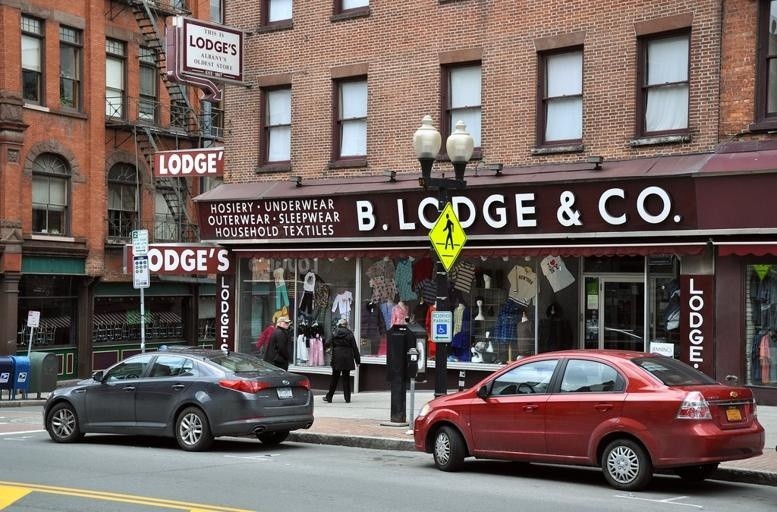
[336,319,349,326]
[277,317,291,322]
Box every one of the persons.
[759,329,774,385]
[380,298,396,329]
[255,323,277,350]
[516,317,534,355]
[392,300,409,325]
[267,317,293,372]
[752,329,765,380]
[322,319,361,403]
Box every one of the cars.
[42,348,314,452]
[413,349,766,491]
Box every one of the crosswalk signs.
[428,201,468,272]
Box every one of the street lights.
[413,114,474,396]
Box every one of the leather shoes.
[322,397,333,403]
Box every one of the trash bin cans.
[0,352,57,400]
